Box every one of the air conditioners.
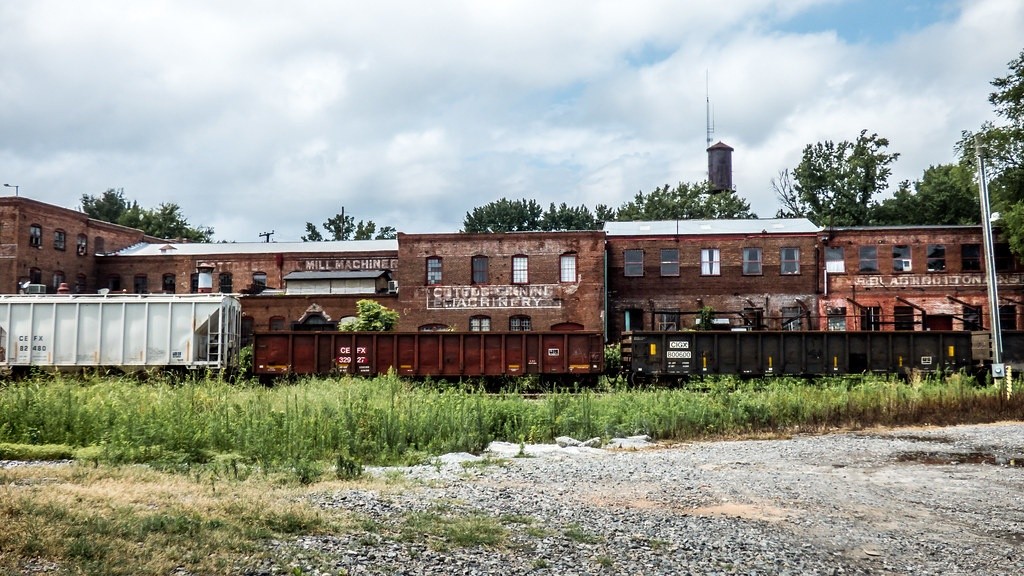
[21,283,45,293]
[388,280,398,292]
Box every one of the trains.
[0,292,1024,392]
